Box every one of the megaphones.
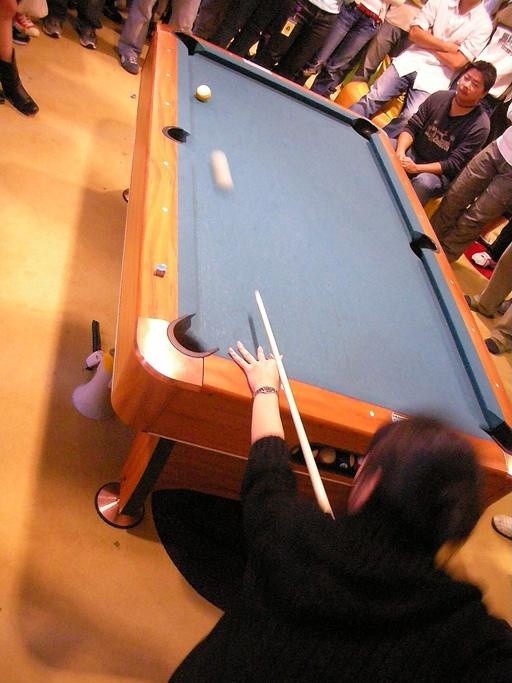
[71,349,115,423]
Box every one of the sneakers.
[492,515,512,539]
[13,10,123,49]
[485,337,511,354]
[472,251,497,269]
[121,53,138,75]
[464,295,480,312]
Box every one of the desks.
[95,22,512,533]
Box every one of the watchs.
[253,385,280,398]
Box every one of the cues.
[255,287,336,521]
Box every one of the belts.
[357,3,382,24]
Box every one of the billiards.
[210,149,231,189]
[195,83,211,102]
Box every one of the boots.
[0,48,39,114]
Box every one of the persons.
[158,338,511,682]
[382,58,498,205]
[1,0,512,152]
[468,217,511,267]
[427,121,510,266]
[463,238,511,357]
